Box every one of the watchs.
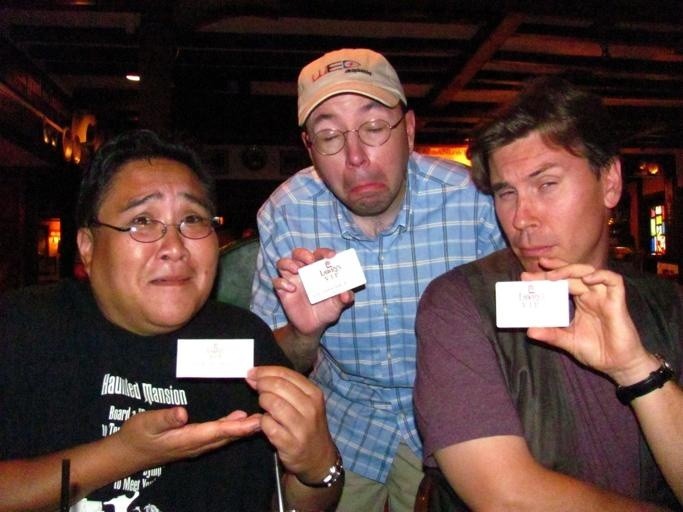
[617,352,672,400]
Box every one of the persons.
[0,128,347,512]
[248,41,509,512]
[209,177,268,309]
[412,77,681,512]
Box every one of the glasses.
[88,214,224,243]
[305,114,404,156]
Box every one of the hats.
[297,47,407,127]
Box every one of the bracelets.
[297,454,347,491]
